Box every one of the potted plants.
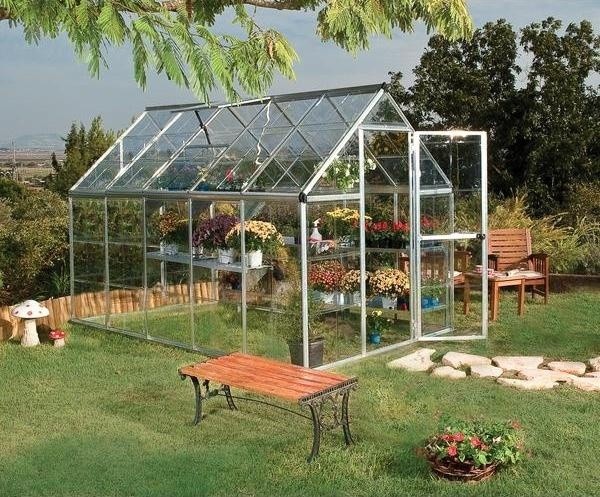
[274,256,331,367]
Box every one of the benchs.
[178,350,359,462]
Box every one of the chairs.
[488,227,548,304]
[398,240,471,317]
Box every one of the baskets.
[426,452,498,483]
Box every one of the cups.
[475,265,484,273]
[487,268,494,278]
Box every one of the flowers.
[314,152,374,187]
[432,413,525,471]
[156,161,301,312]
[305,208,453,343]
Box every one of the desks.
[465,269,524,319]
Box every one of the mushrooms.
[47,330,67,347]
[12,299,50,349]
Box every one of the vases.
[420,441,496,484]
